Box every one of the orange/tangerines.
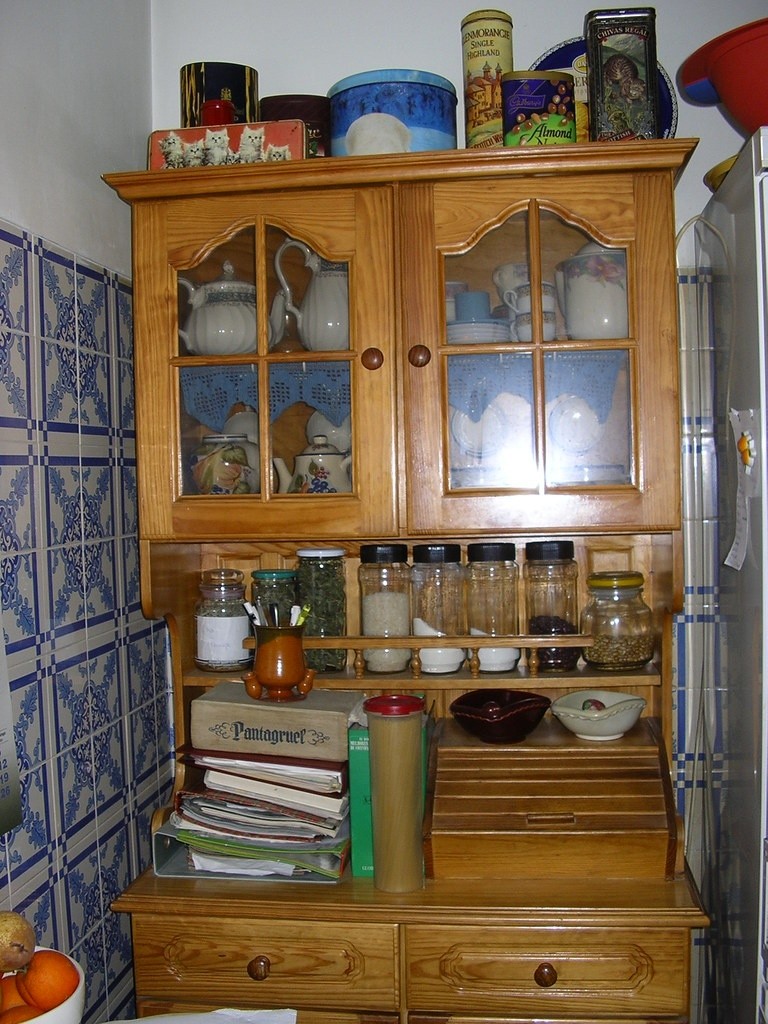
[0,951,81,1024]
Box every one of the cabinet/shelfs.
[100,134,711,1024]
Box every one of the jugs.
[554,240,628,340]
[275,237,350,352]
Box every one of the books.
[153,683,363,883]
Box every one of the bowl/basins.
[571,464,625,483]
[327,68,459,158]
[551,689,647,741]
[449,689,551,745]
[451,465,511,486]
[675,14,767,130]
[0,944,84,1024]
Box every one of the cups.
[509,312,557,343]
[503,281,555,316]
[199,100,235,126]
[260,94,332,160]
[492,262,530,303]
[501,71,578,147]
[179,61,259,128]
[460,9,514,149]
[454,290,491,320]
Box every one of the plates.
[447,318,510,344]
[548,395,605,454]
[552,479,629,486]
[451,400,511,458]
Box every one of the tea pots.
[271,435,352,494]
[177,260,287,356]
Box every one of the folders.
[152,821,337,885]
[175,743,349,798]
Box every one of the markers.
[244,602,261,626]
[289,606,300,627]
[270,603,279,628]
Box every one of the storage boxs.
[189,679,365,763]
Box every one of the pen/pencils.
[296,603,311,626]
[257,597,269,626]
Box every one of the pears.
[0,911,36,973]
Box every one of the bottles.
[409,543,466,675]
[193,568,255,673]
[295,548,349,672]
[522,540,580,674]
[357,543,410,673]
[249,567,296,627]
[187,432,261,496]
[579,569,656,672]
[466,542,520,673]
